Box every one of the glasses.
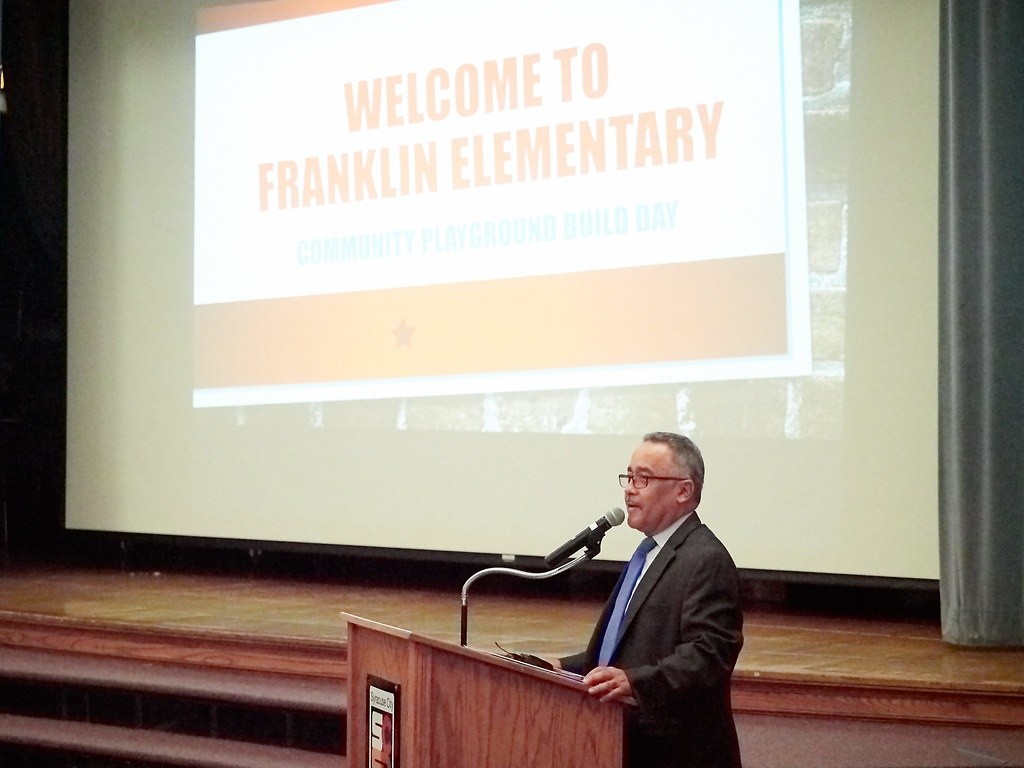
[619,474,688,489]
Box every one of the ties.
[599,537,658,667]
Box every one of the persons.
[503,431,747,768]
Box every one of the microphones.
[544,507,625,568]
